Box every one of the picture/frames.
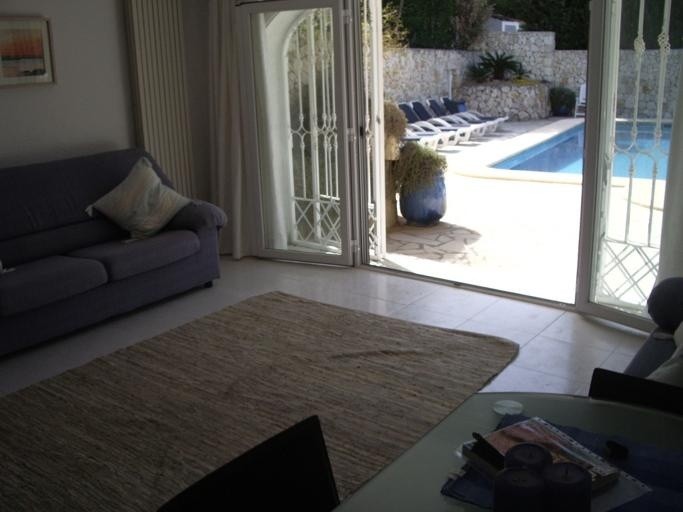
[0,13,59,92]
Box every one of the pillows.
[85,155,195,246]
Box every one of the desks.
[333,392,683,510]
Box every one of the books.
[461,416,620,494]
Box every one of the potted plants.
[383,98,407,161]
[548,86,576,117]
[395,142,447,227]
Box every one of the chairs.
[575,83,588,117]
[155,413,341,510]
[589,368,682,414]
[397,97,509,152]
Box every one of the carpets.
[0,290,520,511]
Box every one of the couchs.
[1,145,229,364]
[622,276,683,388]
